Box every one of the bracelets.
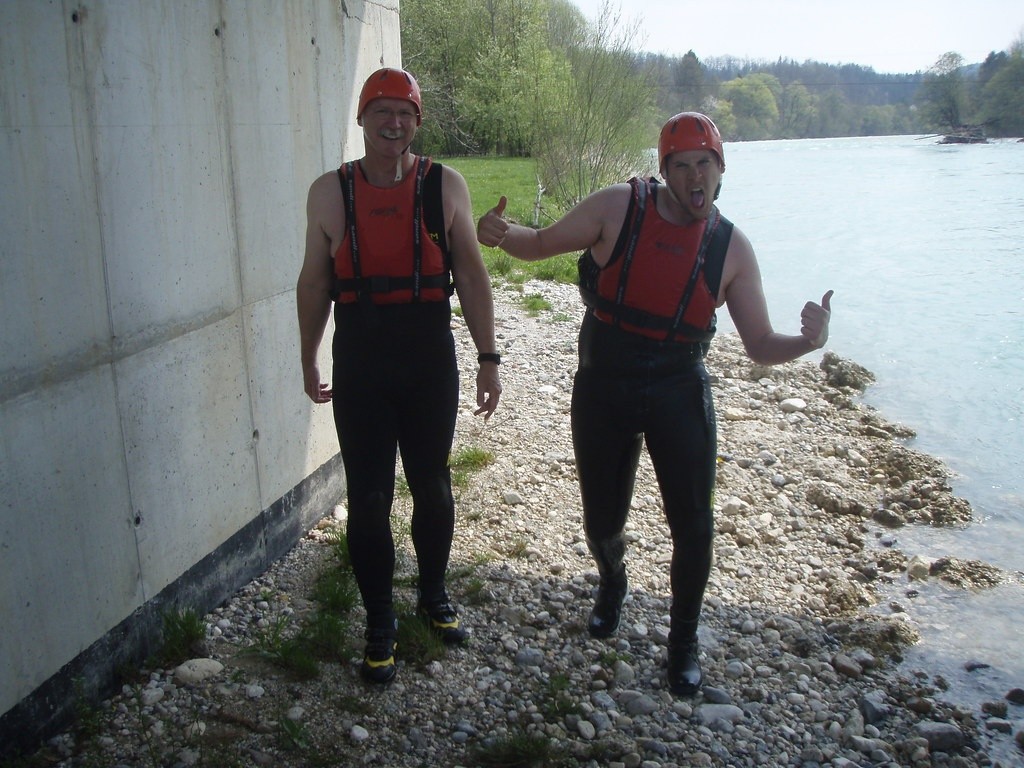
[477,354,501,365]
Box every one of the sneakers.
[416,601,467,645]
[362,630,394,684]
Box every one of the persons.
[485,112,834,698]
[298,69,504,690]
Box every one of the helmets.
[356,67,422,126]
[658,111,725,171]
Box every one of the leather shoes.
[666,630,702,694]
[589,563,628,634]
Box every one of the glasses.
[365,105,420,122]
